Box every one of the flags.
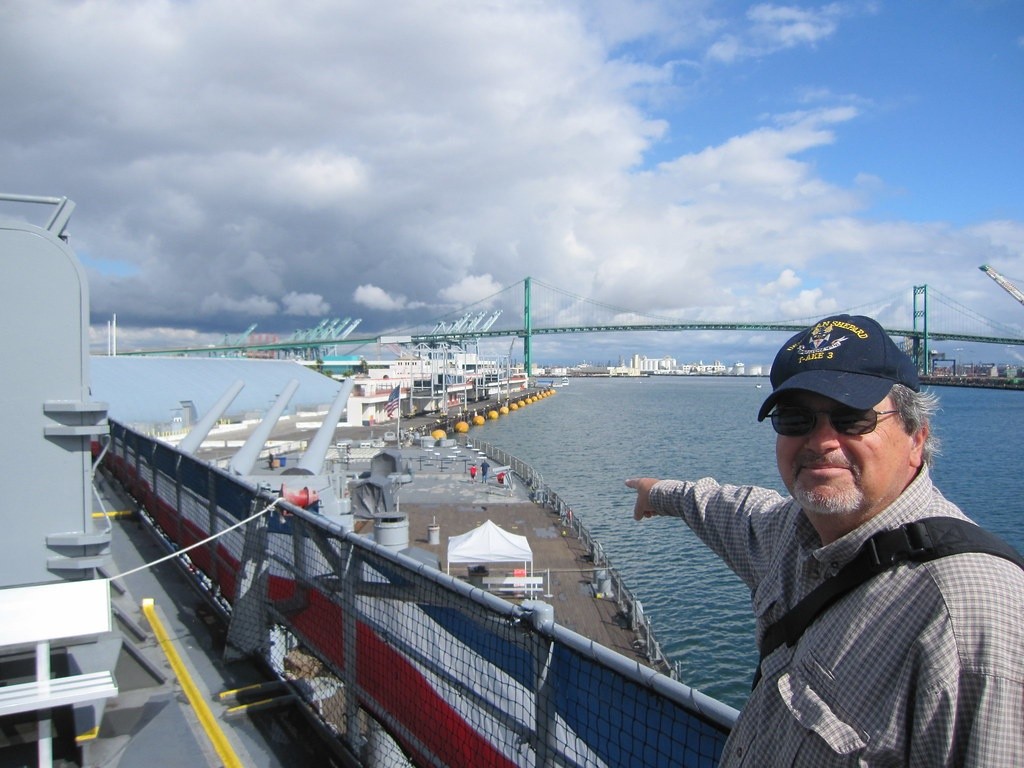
[384,386,399,417]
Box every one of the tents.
[447,519,533,601]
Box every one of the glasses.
[764,403,900,436]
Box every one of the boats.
[561,377,569,386]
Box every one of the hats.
[757,314,921,422]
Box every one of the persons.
[268,453,274,470]
[480,460,491,483]
[625,312,1024,768]
[470,464,477,484]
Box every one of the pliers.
[216,675,340,722]
